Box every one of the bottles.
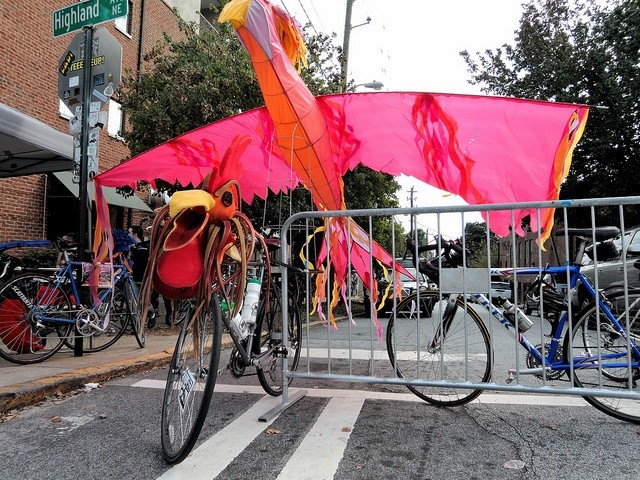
[222,299,250,341]
[242,276,262,326]
[497,295,533,332]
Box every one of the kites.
[84,1,612,330]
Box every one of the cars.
[524,273,568,314]
[564,228,640,334]
[363,269,433,317]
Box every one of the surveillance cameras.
[366,16,371,22]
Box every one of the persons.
[125,224,149,287]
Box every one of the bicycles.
[2,237,150,364]
[161,240,301,464]
[584,259,640,381]
[165,258,283,326]
[387,225,640,424]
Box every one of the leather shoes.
[147,312,156,329]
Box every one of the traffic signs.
[345,80,386,93]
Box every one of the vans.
[384,258,439,307]
[491,281,511,304]
